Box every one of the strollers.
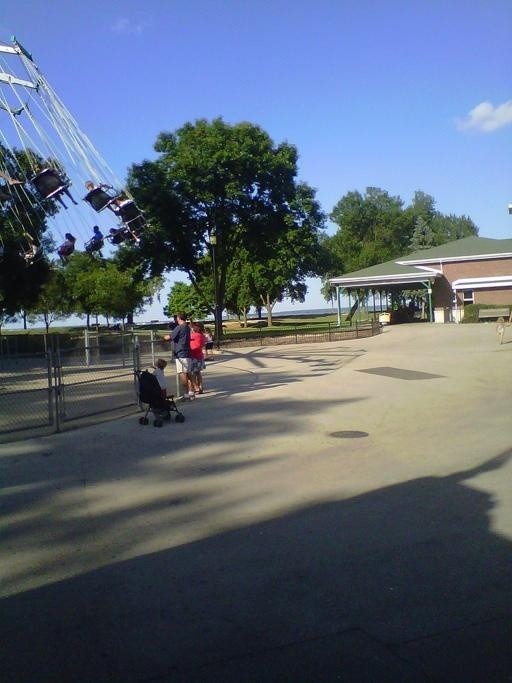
[136,370,185,427]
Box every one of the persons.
[0,164,140,262]
[164,311,195,403]
[190,322,206,395]
[204,328,214,361]
[151,359,167,399]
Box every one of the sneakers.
[176,394,195,402]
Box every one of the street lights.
[209,229,218,348]
